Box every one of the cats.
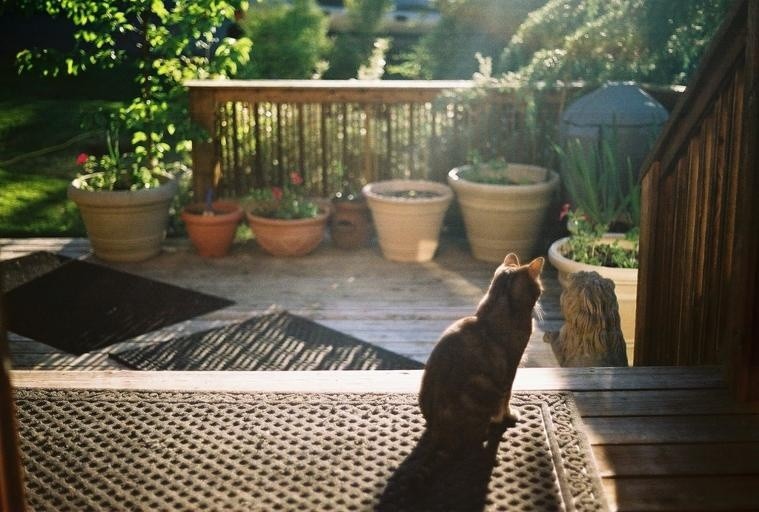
[418,252,545,447]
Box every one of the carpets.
[0,252,235,357]
[108,308,426,371]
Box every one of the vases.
[361,175,454,264]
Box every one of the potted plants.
[446,143,561,264]
[328,175,373,250]
[181,184,244,260]
[67,112,178,264]
[546,108,646,366]
[246,168,333,256]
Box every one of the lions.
[543,271,628,367]
